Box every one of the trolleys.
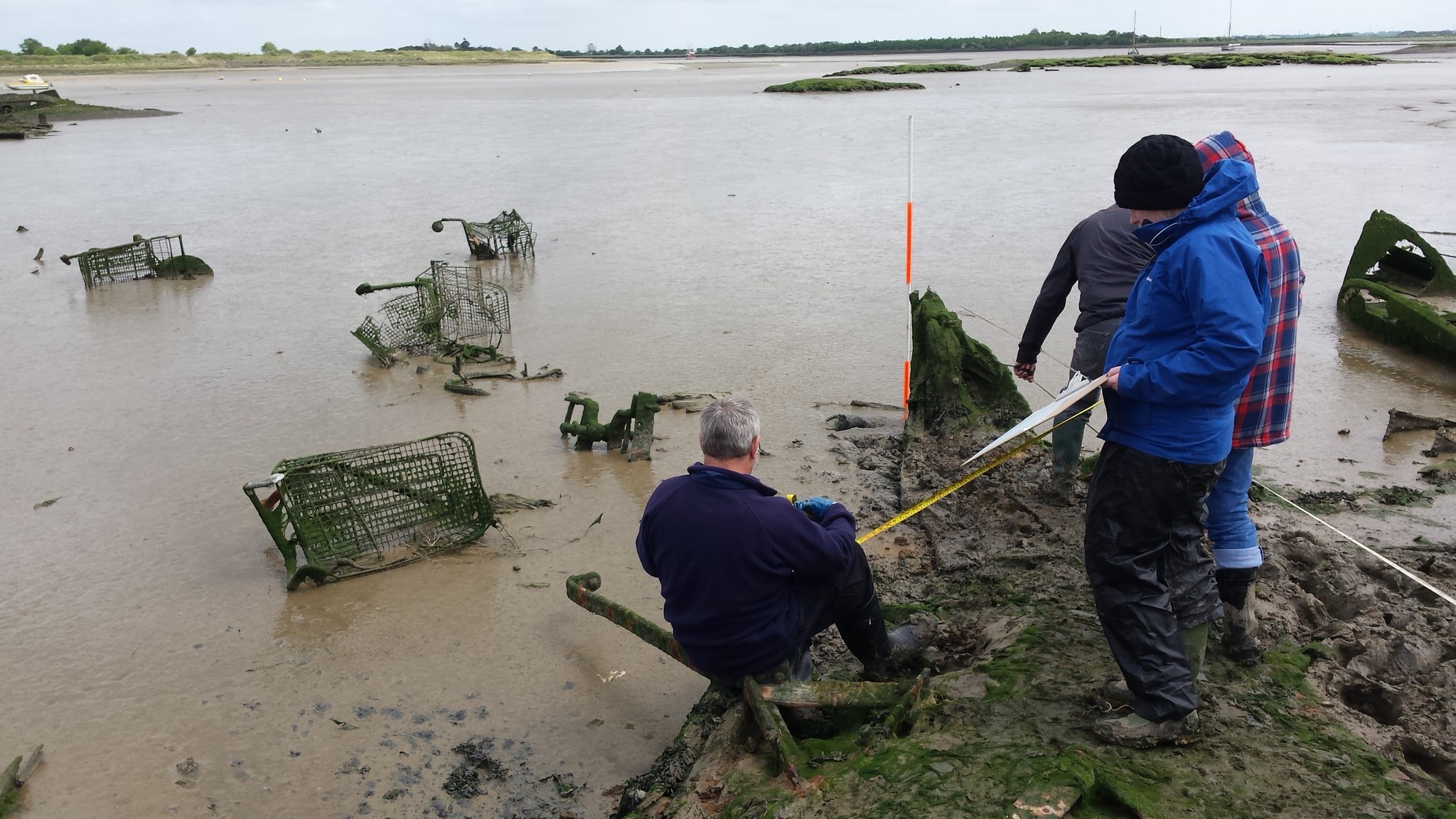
[60,229,188,292]
[432,211,540,260]
[354,264,511,362]
[243,431,494,592]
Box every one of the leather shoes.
[1104,681,1137,707]
[1094,709,1201,748]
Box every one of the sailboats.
[1221,0,1244,51]
[1127,9,1139,55]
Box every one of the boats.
[6,75,49,90]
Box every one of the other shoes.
[1042,483,1071,507]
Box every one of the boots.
[1216,576,1262,666]
[791,651,814,725]
[835,588,937,682]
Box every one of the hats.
[1113,134,1204,210]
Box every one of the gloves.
[794,496,834,520]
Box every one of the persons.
[1013,199,1164,503]
[634,395,939,683]
[1084,132,1273,752]
[1190,129,1307,667]
